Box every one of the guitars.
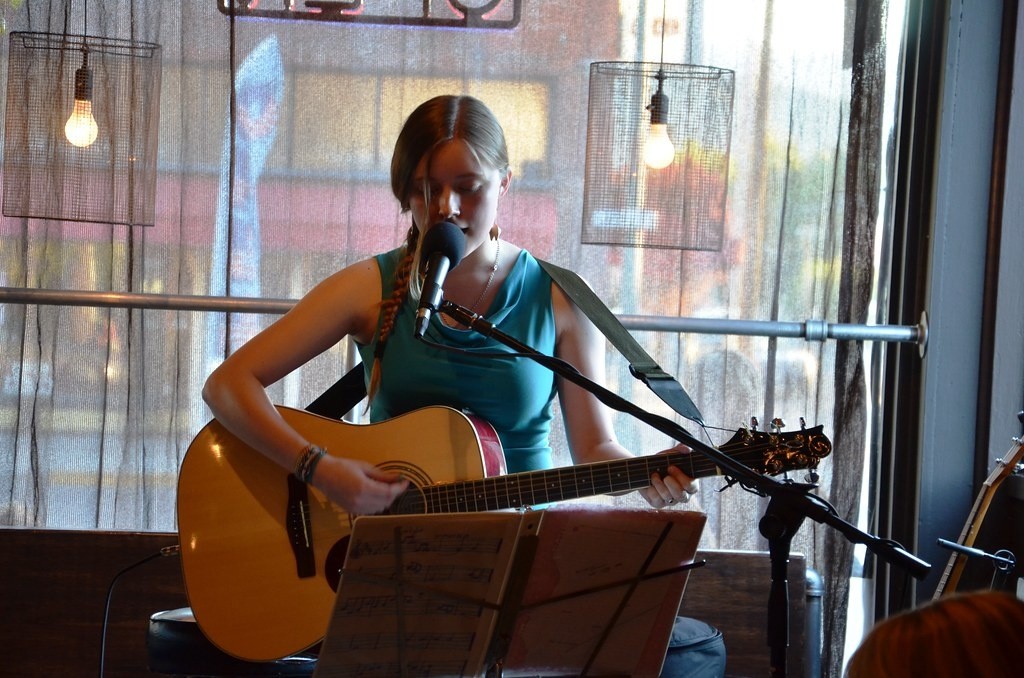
[177,403,832,663]
[930,410,1024,600]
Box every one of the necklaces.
[439,241,500,329]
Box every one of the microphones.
[414,221,466,337]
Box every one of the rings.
[667,498,674,504]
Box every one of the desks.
[146,608,725,678]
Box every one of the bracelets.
[295,444,326,485]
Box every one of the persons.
[201,95,700,515]
